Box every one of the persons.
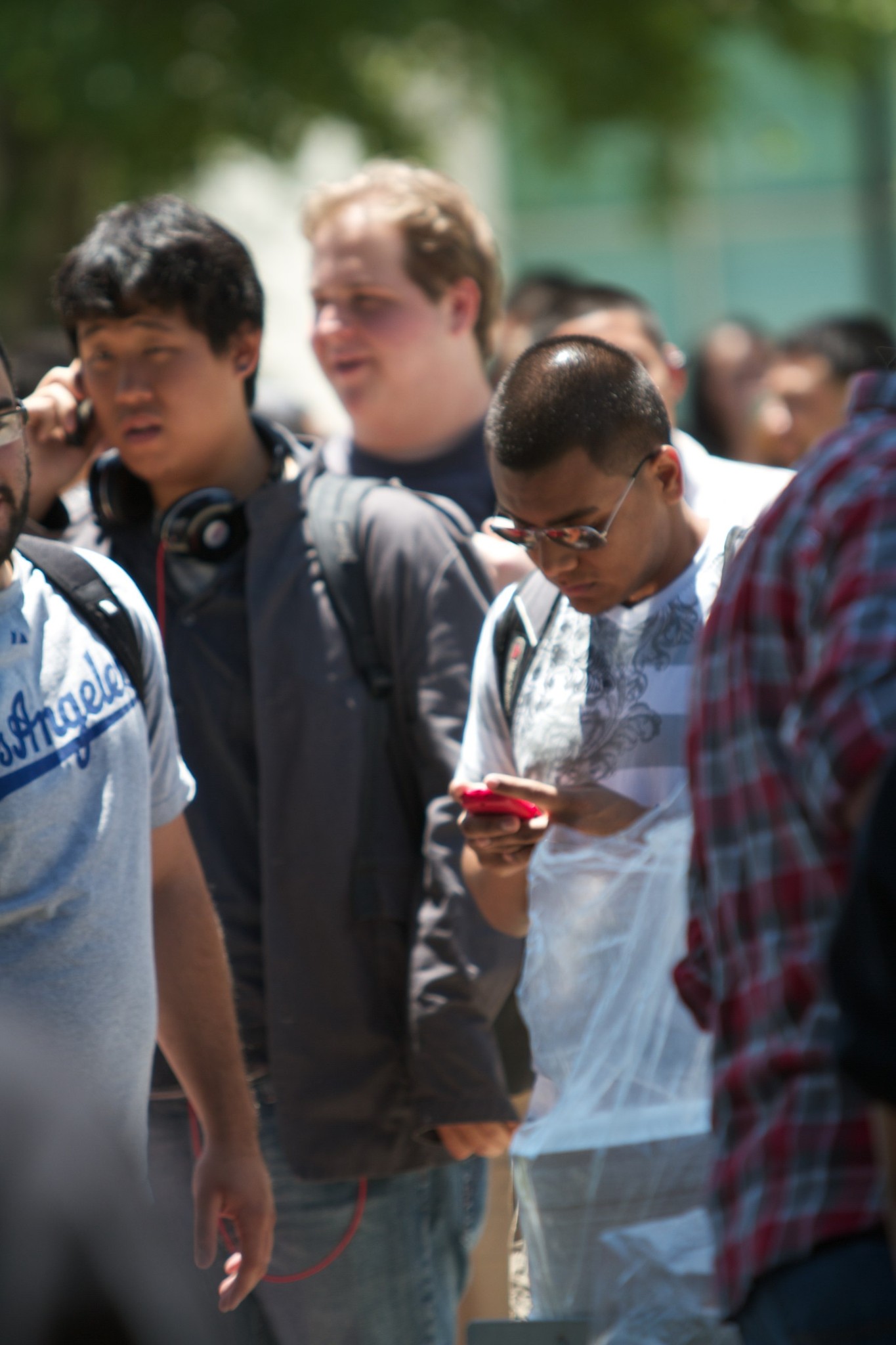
[0,351,276,1345]
[15,163,896,1345]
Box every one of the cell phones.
[67,397,96,452]
[458,788,542,823]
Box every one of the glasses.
[0,398,27,445]
[488,453,657,552]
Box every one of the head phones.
[88,415,308,564]
